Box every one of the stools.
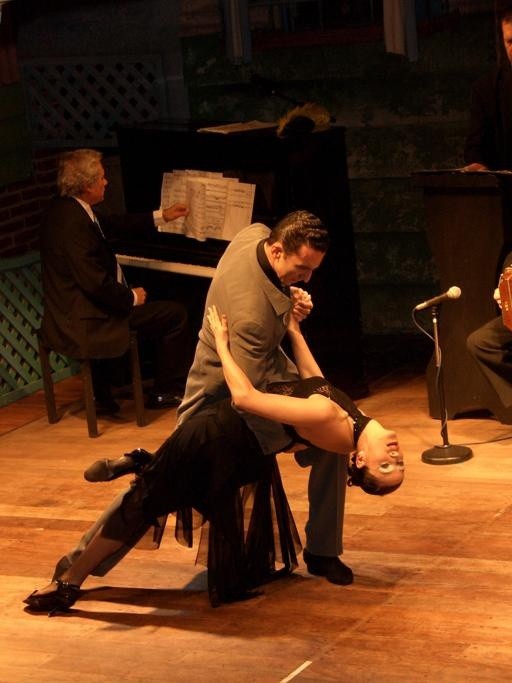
[37,329,145,438]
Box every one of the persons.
[456,11,512,171]
[38,147,193,410]
[466,249,512,428]
[22,289,405,617]
[50,209,353,585]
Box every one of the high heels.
[22,579,80,617]
[84,448,154,482]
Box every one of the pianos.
[114,127,370,408]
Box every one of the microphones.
[414,286,462,310]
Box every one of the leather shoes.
[147,394,181,409]
[303,548,353,586]
[95,396,121,415]
